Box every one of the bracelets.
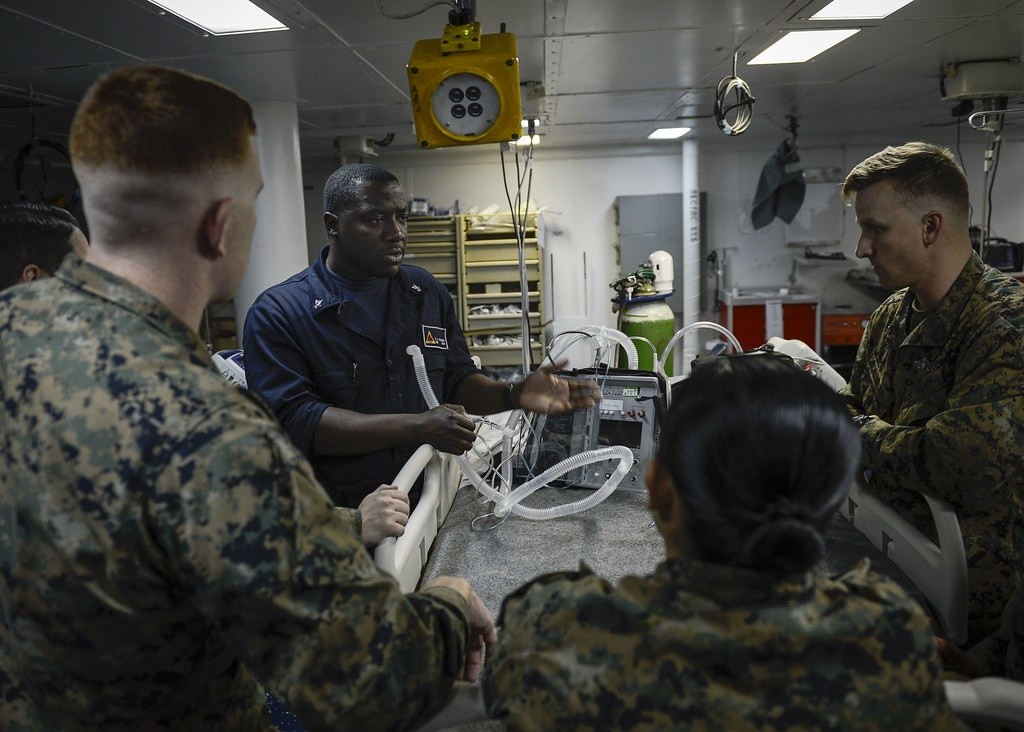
[504,383,516,411]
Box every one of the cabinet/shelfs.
[719,286,823,357]
[400,210,546,366]
[821,307,879,367]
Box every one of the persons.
[0,69,494,731]
[843,142,1024,684]
[242,164,604,509]
[481,354,968,732]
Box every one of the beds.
[374,407,971,732]
